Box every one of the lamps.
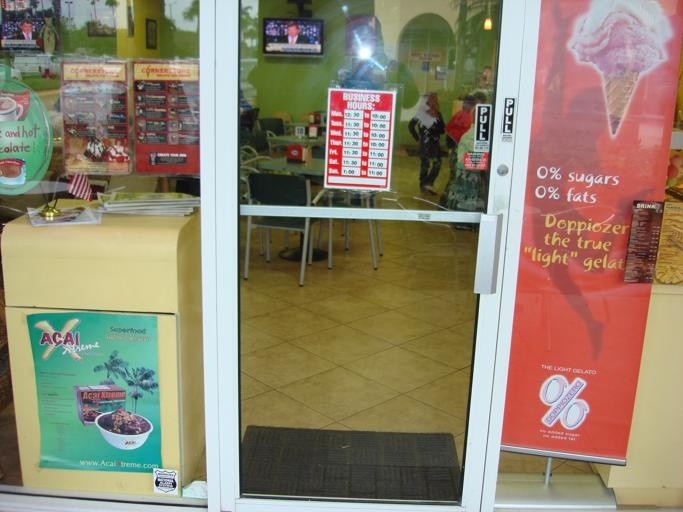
[484,1,493,30]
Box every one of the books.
[92,191,201,217]
[26,205,100,226]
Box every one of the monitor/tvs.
[262,16,324,60]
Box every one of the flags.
[67,174,92,202]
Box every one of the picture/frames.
[146,18,157,49]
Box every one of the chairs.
[176,107,383,287]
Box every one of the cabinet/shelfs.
[0,199,206,496]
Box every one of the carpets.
[241,425,460,503]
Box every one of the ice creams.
[567,1,674,135]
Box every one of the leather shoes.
[423,185,437,196]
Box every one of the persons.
[15,19,39,40]
[473,91,486,122]
[49,153,75,201]
[409,93,445,195]
[276,24,308,55]
[438,97,481,209]
